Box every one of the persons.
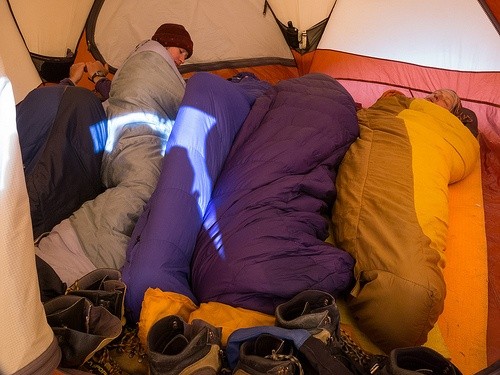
[152,23,193,68]
[386,89,462,116]
[58,61,111,102]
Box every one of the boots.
[389,345,463,375]
[233,333,306,375]
[146,315,230,375]
[275,290,388,375]
[43,296,130,375]
[65,268,150,374]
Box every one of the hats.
[151,23,193,59]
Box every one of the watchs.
[88,71,107,83]
